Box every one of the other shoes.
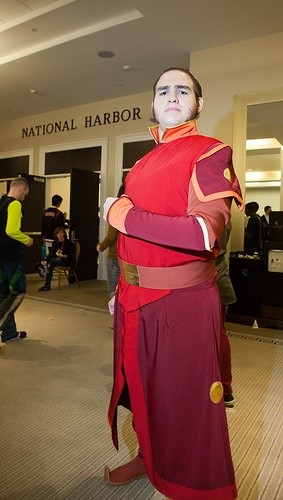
[38,285,51,292]
[103,455,148,486]
[2,331,26,342]
[224,394,236,405]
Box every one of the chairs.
[48,240,82,292]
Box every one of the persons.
[104,67,243,500]
[0,177,33,351]
[96,184,125,329]
[34,227,76,292]
[244,202,271,254]
[37,194,67,277]
[214,220,237,405]
